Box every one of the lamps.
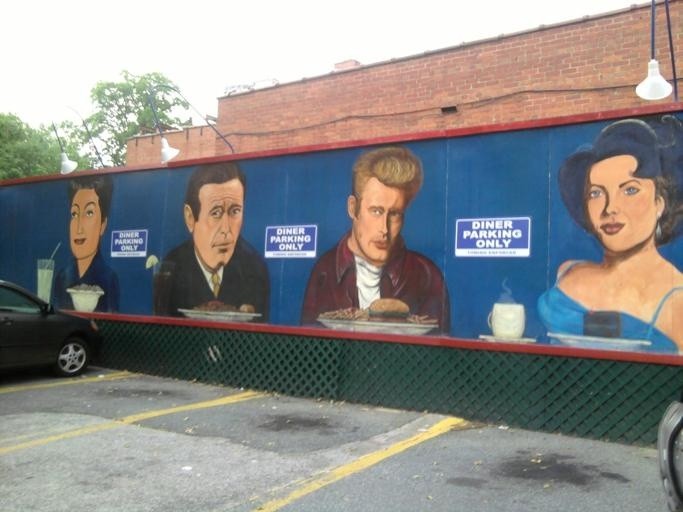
[148,85,235,164]
[635,0,678,103]
[52,107,105,176]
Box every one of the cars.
[0,279,102,377]
[657,400,682,511]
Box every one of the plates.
[546,332,653,353]
[176,306,263,325]
[477,335,536,345]
[316,318,440,336]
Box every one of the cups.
[36,258,55,313]
[487,303,527,340]
[65,288,105,314]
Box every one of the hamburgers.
[367,298,411,323]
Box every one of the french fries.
[319,307,439,322]
